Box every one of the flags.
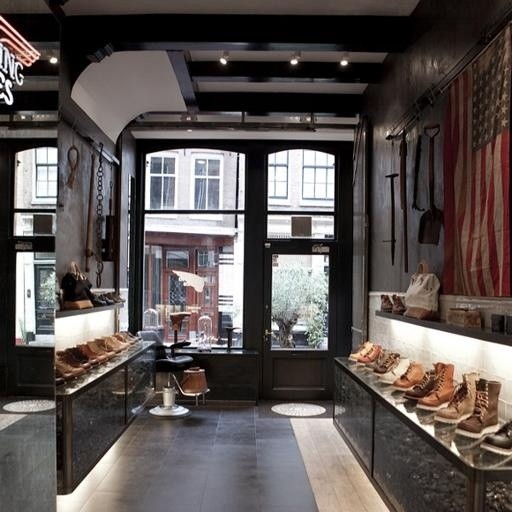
[441,22,512,297]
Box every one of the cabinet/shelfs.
[336,309,512,512]
[54,302,154,493]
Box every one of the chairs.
[137,330,194,417]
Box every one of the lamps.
[219,50,231,66]
[42,46,57,64]
[289,50,303,67]
[340,52,351,66]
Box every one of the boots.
[391,294,405,314]
[380,294,392,312]
[94,292,127,306]
[169,310,192,331]
[349,341,511,459]
[54,331,148,389]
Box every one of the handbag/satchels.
[60,259,93,302]
[405,263,440,319]
